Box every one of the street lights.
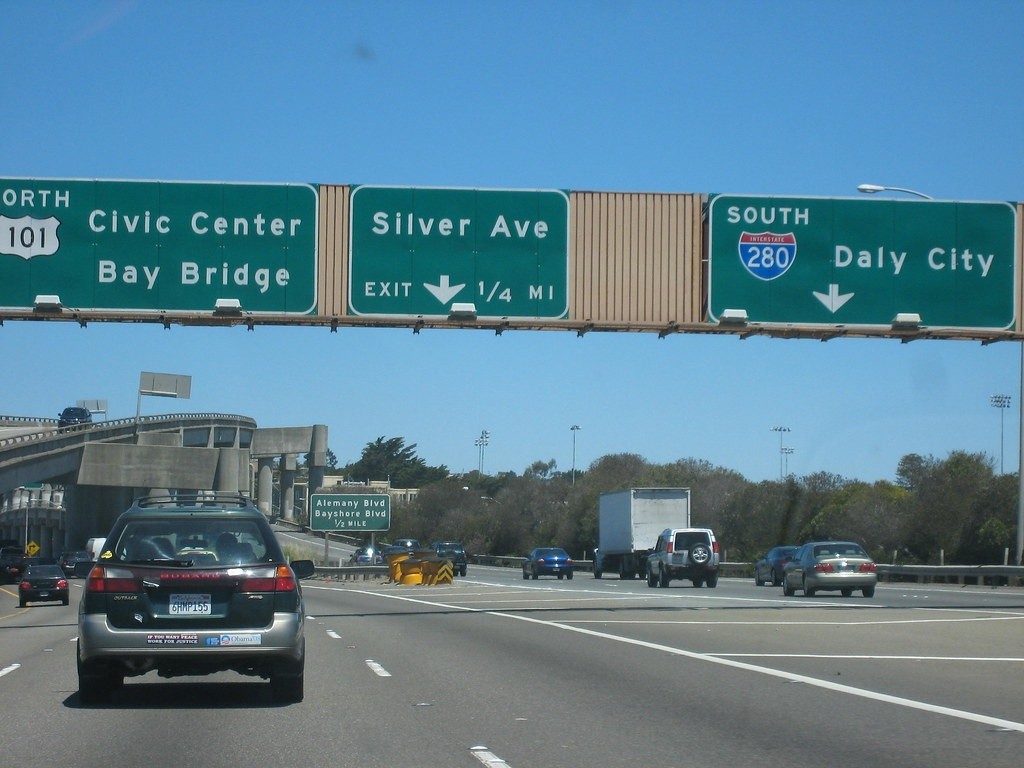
[571,424,582,485]
[16,486,34,552]
[771,426,794,482]
[475,429,490,477]
[990,392,1011,475]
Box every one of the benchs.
[816,550,864,558]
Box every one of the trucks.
[593,487,690,580]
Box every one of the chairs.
[141,537,175,560]
[223,543,257,564]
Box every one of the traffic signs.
[708,192,1015,333]
[345,185,572,321]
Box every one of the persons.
[214,533,238,562]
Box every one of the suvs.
[74,490,314,703]
[431,542,467,577]
[58,408,92,432]
[647,527,718,587]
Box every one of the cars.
[783,543,878,597]
[753,546,800,586]
[57,552,94,577]
[350,539,422,566]
[521,548,573,581]
[0,548,26,578]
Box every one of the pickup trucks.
[18,564,70,605]
